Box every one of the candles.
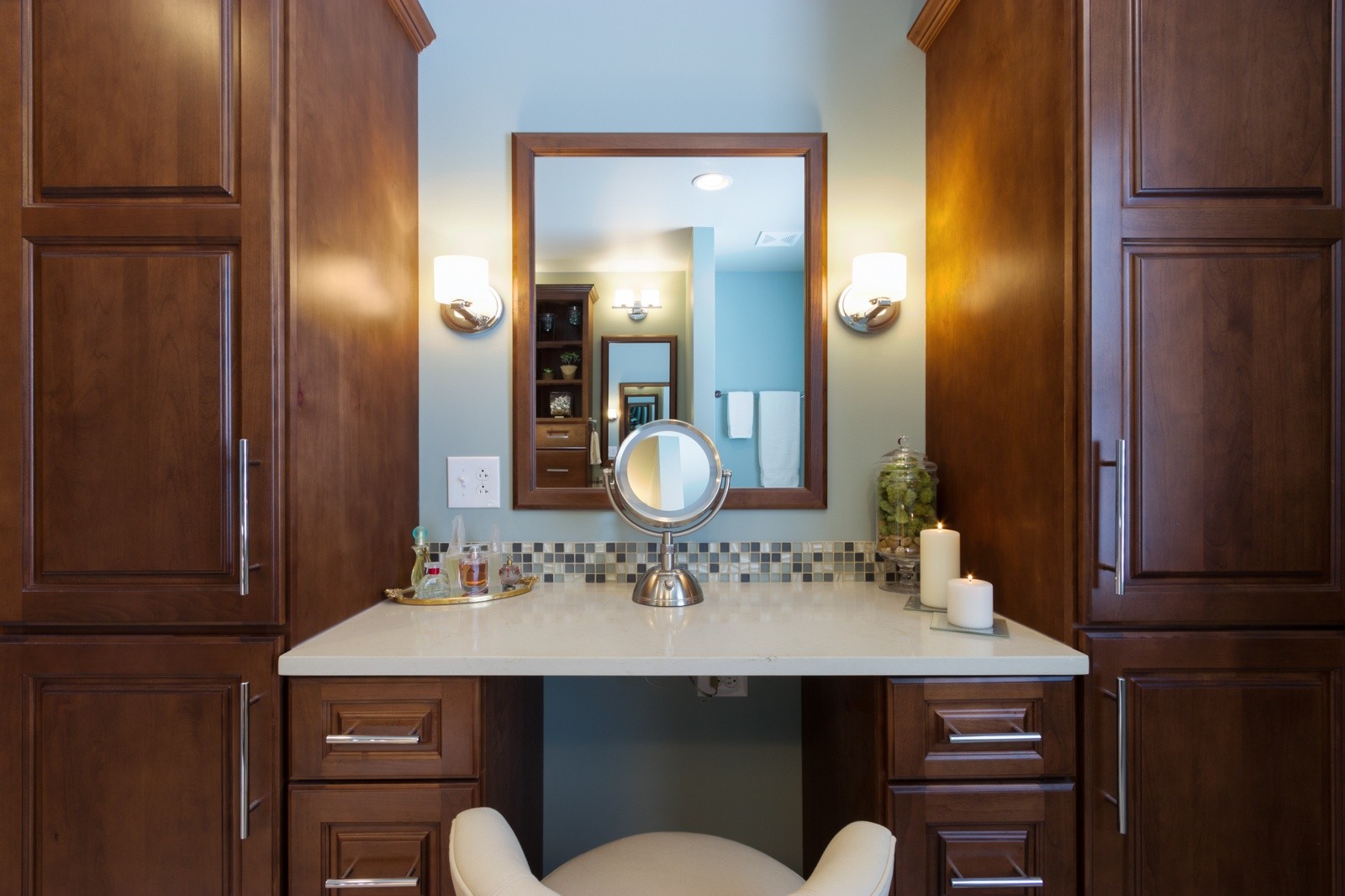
[919,521,962,610]
[947,575,996,629]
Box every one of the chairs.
[447,807,897,896]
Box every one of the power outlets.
[696,674,750,697]
[446,456,501,508]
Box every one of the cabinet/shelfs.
[279,583,1090,896]
[534,283,600,489]
[0,0,438,896]
[902,0,1344,896]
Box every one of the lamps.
[836,252,907,334]
[432,254,504,335]
[612,284,662,321]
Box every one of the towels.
[726,391,754,439]
[591,432,602,465]
[758,391,800,488]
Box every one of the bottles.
[461,545,489,594]
[411,527,431,592]
[488,535,502,594]
[443,529,461,597]
[416,561,450,599]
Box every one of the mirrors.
[601,334,678,467]
[534,155,808,489]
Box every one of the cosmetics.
[441,516,468,589]
[416,560,450,599]
[458,546,491,595]
[411,526,435,591]
[483,523,504,586]
[499,555,522,586]
